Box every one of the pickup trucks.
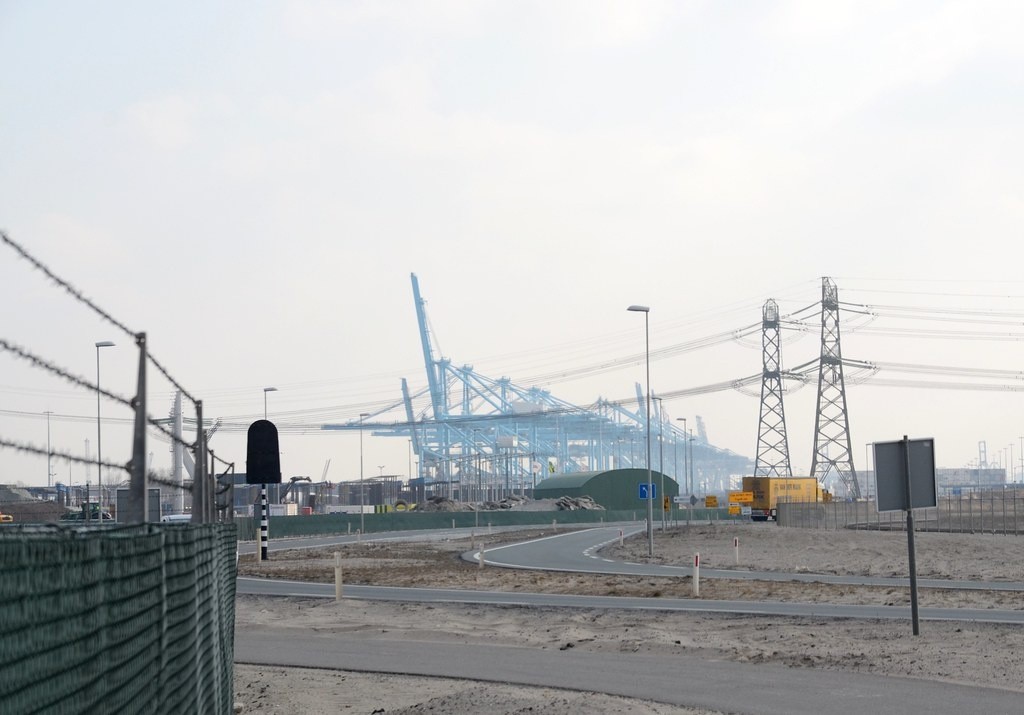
[60,501,112,520]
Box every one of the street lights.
[473,427,484,526]
[263,387,278,420]
[627,304,654,555]
[676,416,687,496]
[407,439,412,480]
[359,412,371,534]
[651,395,666,532]
[95,340,115,524]
[43,410,54,487]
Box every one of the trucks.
[743,475,834,521]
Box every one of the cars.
[0,510,14,522]
